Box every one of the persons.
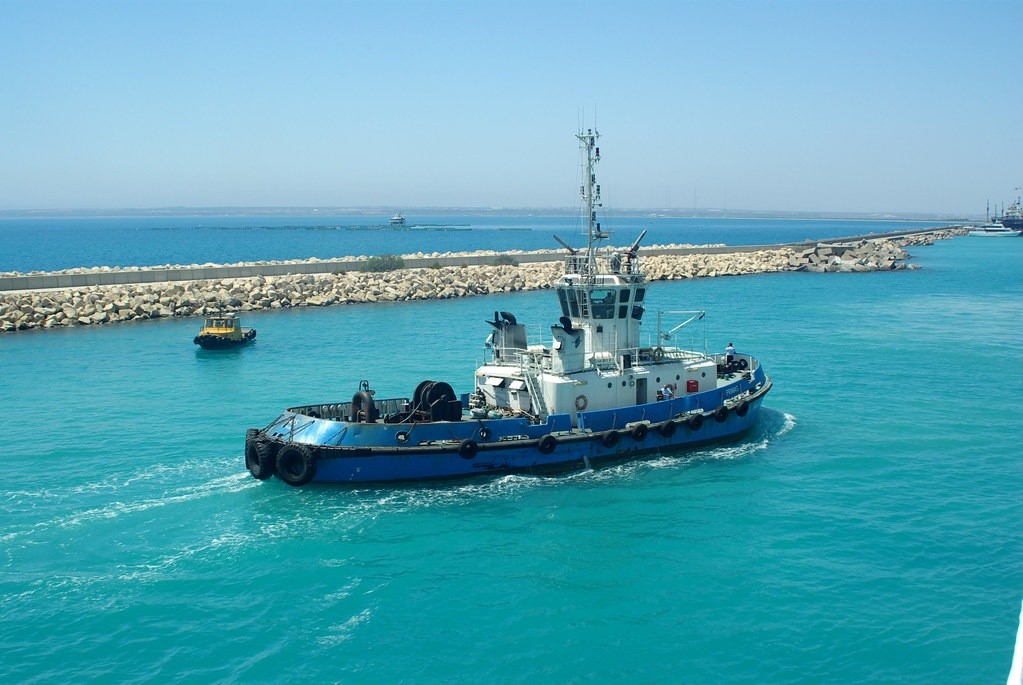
[657,389,663,402]
[724,343,737,365]
[660,385,676,400]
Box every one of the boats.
[966,194,1023,238]
[193,300,256,350]
[243,101,774,485]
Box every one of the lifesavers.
[652,347,665,362]
[661,420,676,437]
[632,423,648,440]
[689,413,704,431]
[575,394,589,411]
[245,438,274,482]
[274,442,314,485]
[715,405,728,422]
[245,427,259,469]
[603,431,622,448]
[538,434,556,455]
[459,439,479,459]
[736,399,750,417]
